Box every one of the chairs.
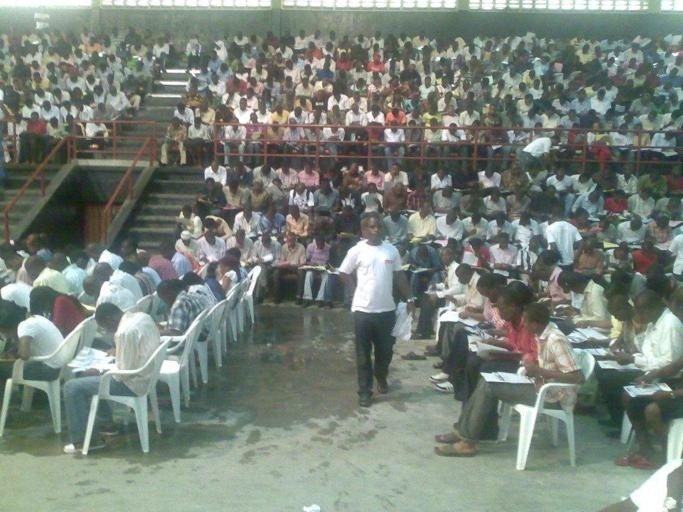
[152,289,161,315]
[244,264,261,324]
[157,317,201,424]
[63,314,97,381]
[81,337,172,456]
[198,262,209,280]
[235,277,250,334]
[159,308,208,389]
[219,281,242,352]
[77,291,96,304]
[501,347,597,471]
[123,304,136,313]
[195,298,229,385]
[137,294,153,313]
[619,412,631,444]
[0,319,91,438]
[667,417,683,464]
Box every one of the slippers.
[432,361,443,368]
[401,351,426,360]
[434,444,475,458]
[435,433,460,443]
[424,349,440,356]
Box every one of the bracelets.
[407,296,417,305]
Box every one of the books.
[470,341,523,361]
[479,371,534,384]
[621,383,673,398]
[438,310,478,327]
[465,334,485,354]
[596,360,641,371]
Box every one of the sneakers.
[598,415,617,427]
[432,381,454,393]
[614,453,636,467]
[429,372,451,382]
[63,433,107,454]
[359,394,371,407]
[376,377,388,394]
[632,456,655,470]
[99,426,120,436]
[605,427,620,439]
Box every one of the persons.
[411,244,442,296]
[324,244,354,309]
[176,203,203,233]
[454,290,536,444]
[603,456,683,512]
[301,235,333,308]
[153,279,210,343]
[487,211,514,244]
[125,254,163,289]
[138,250,179,281]
[614,353,682,470]
[286,205,309,238]
[430,268,484,369]
[333,206,361,246]
[203,215,232,238]
[429,275,506,393]
[517,237,540,281]
[462,239,489,271]
[463,212,489,240]
[226,228,255,271]
[0,225,79,383]
[226,247,248,281]
[95,262,145,301]
[338,211,417,408]
[272,232,307,305]
[176,231,199,253]
[487,232,521,275]
[0,28,180,162]
[219,255,242,293]
[454,281,535,402]
[118,259,154,296]
[197,229,228,267]
[410,247,467,340]
[542,211,683,360]
[183,271,219,307]
[425,263,484,357]
[511,211,539,246]
[434,303,580,457]
[233,206,261,236]
[158,241,192,280]
[407,202,436,245]
[177,280,218,309]
[82,276,137,313]
[437,209,464,241]
[62,302,161,454]
[252,234,281,303]
[382,205,409,240]
